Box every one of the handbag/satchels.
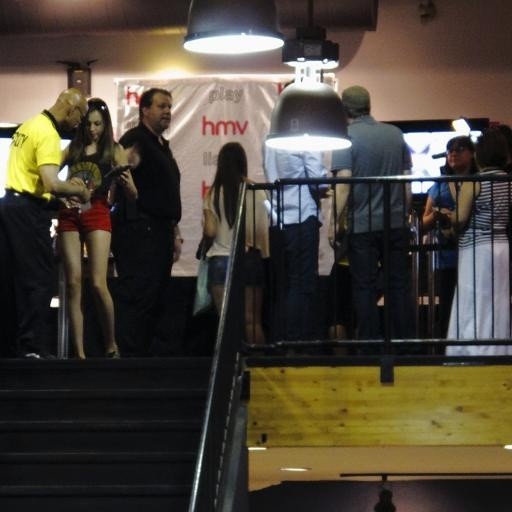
[192,237,214,316]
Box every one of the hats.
[341,86,370,110]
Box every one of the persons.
[332,86,417,338]
[57,96,142,363]
[198,141,272,349]
[444,130,512,366]
[109,86,185,360]
[260,132,331,343]
[0,88,93,360]
[423,132,477,339]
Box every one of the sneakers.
[13,346,56,361]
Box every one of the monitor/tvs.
[378,117,490,202]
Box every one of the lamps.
[181,0,353,153]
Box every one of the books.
[103,164,132,179]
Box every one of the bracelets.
[79,187,86,196]
[174,234,184,242]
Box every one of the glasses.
[445,143,467,155]
